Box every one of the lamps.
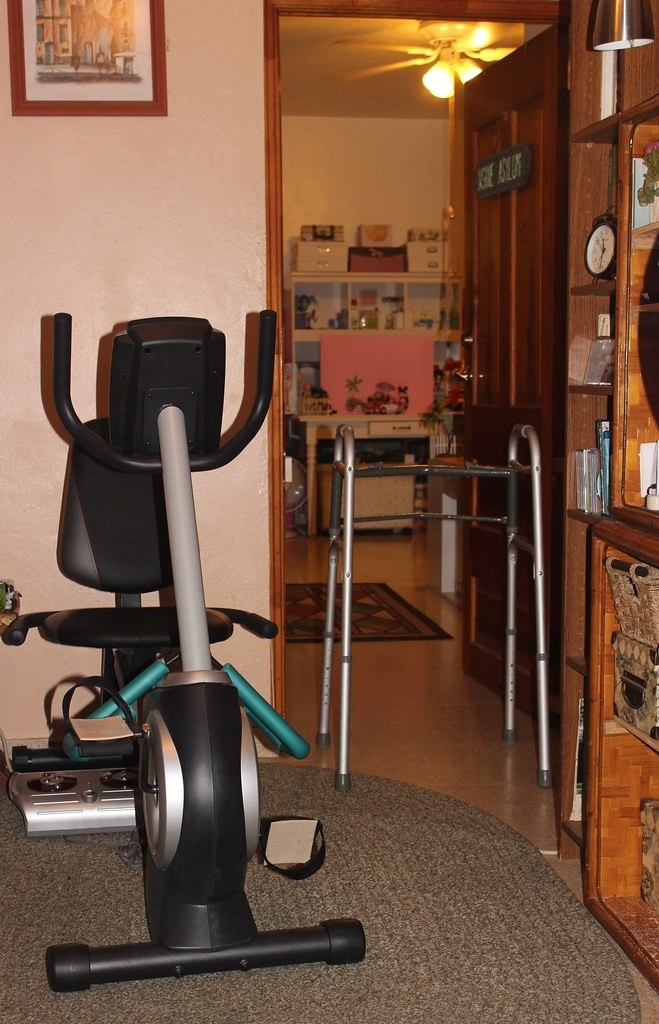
[409,18,517,99]
[586,0,656,52]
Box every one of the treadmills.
[1,307,373,995]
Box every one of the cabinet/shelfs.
[285,274,463,416]
[558,0,658,991]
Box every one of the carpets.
[283,581,454,644]
[0,766,642,1024]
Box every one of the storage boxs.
[300,225,345,241]
[403,242,451,272]
[348,247,405,274]
[610,631,658,741]
[408,228,450,242]
[603,556,659,649]
[293,241,348,273]
[358,224,392,248]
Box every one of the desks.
[295,411,469,539]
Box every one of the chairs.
[0,418,279,742]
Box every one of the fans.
[330,40,517,81]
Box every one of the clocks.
[584,203,617,284]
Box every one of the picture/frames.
[6,0,168,117]
